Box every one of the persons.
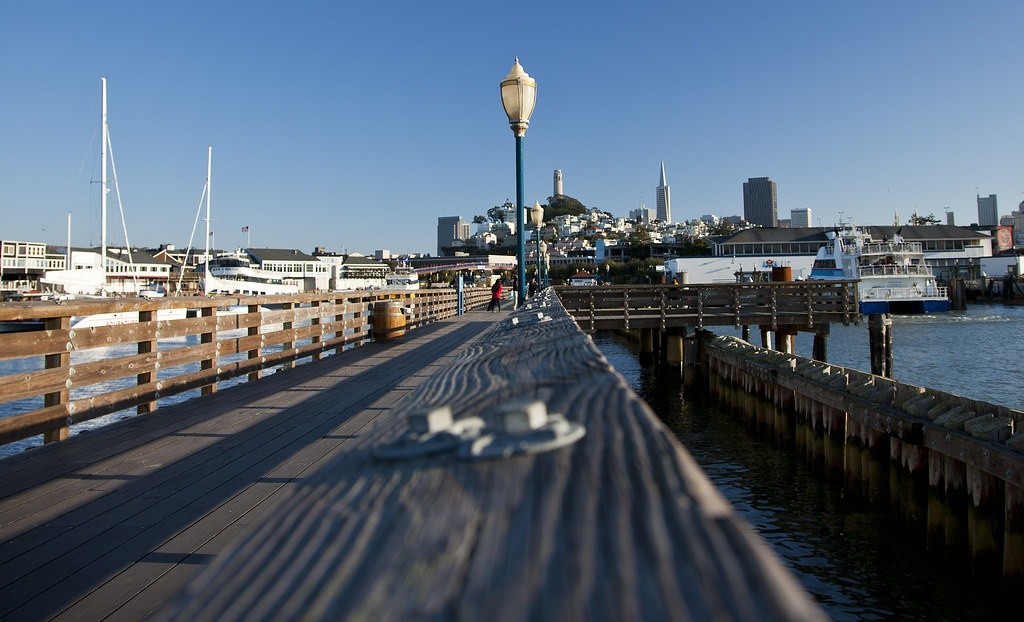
[513,275,518,310]
[528,278,538,297]
[491,279,503,313]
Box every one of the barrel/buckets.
[374,302,405,344]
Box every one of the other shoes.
[499,311,502,313]
[492,311,495,313]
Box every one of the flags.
[210,232,213,236]
[241,226,248,232]
